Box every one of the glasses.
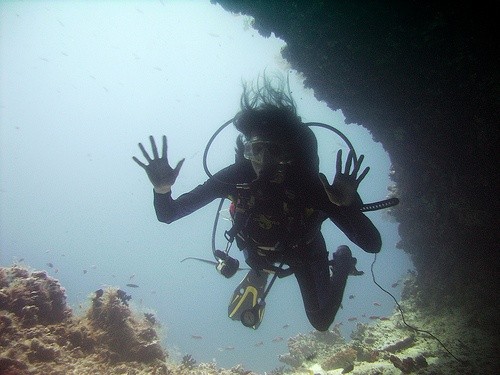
[242,139,294,164]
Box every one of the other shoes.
[335,244,364,276]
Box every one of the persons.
[131,71,383,333]
